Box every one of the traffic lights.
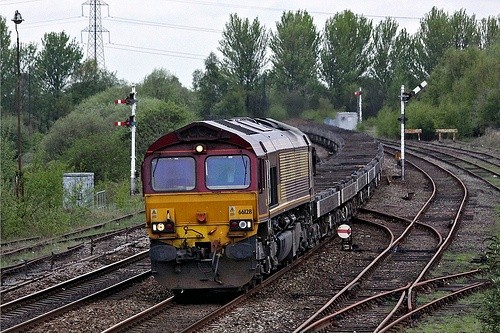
[126,120,131,126]
[354,91,360,96]
[125,98,130,104]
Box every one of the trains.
[137,115,385,304]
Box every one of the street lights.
[11,9,26,201]
